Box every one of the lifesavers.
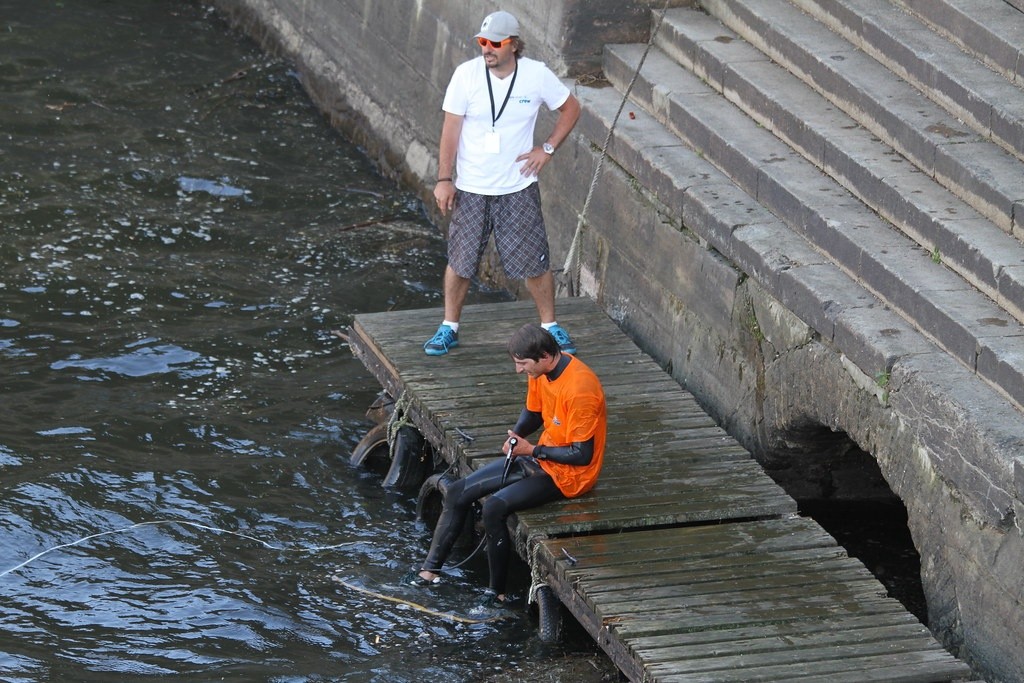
[351,420,424,490]
[524,584,565,643]
[416,473,472,550]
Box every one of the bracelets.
[437,178,453,182]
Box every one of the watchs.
[542,143,555,156]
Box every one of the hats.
[472,11,519,42]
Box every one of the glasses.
[477,36,513,50]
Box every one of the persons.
[424,11,581,356]
[415,324,607,608]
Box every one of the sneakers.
[547,324,576,355]
[470,587,506,615]
[423,324,460,356]
[408,574,442,589]
